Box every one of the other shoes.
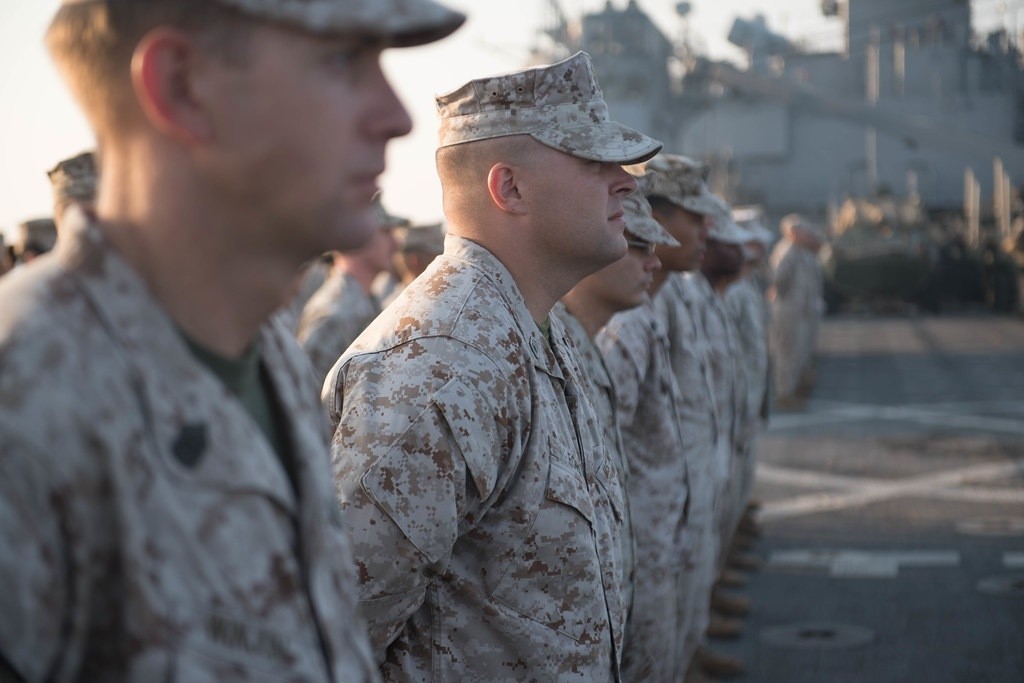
[686,493,764,682]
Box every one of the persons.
[550,155,826,683]
[0,146,446,391]
[1,0,465,682]
[317,50,663,682]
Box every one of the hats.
[779,215,807,233]
[233,1,466,48]
[705,193,772,245]
[435,51,663,166]
[392,223,444,256]
[620,178,681,248]
[15,220,58,254]
[367,195,409,228]
[621,153,729,216]
[47,153,98,204]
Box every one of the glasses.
[627,239,657,256]
